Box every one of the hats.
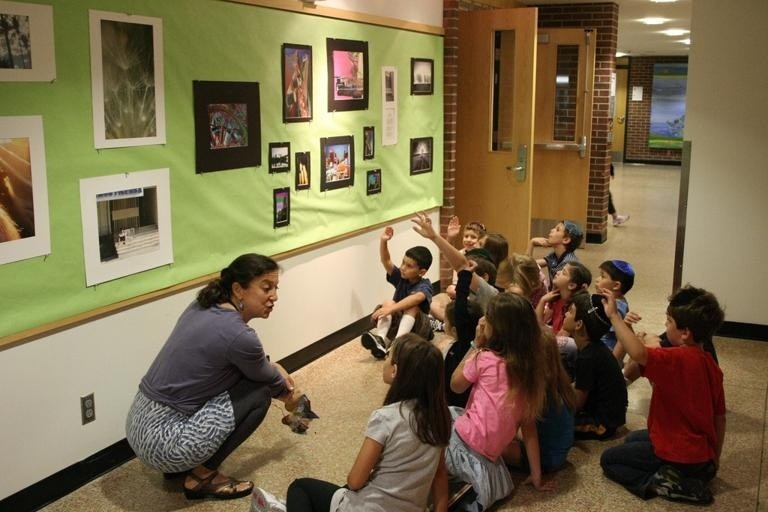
[612,258,634,276]
[590,293,612,327]
[466,248,495,263]
[564,219,584,237]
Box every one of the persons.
[609,163,630,229]
[360,211,727,512]
[251,330,451,511]
[126,253,302,501]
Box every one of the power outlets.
[80,392,95,425]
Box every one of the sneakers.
[428,481,473,511]
[426,314,445,333]
[651,460,717,505]
[251,487,287,511]
[362,331,396,360]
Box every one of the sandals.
[164,473,186,478]
[184,471,253,500]
